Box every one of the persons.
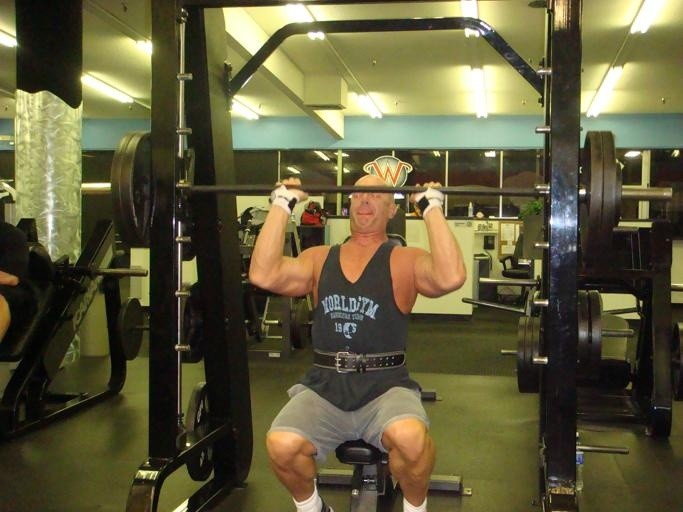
[0,270,20,344]
[247,176,467,512]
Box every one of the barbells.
[80,132,675,249]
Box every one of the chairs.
[498,236,535,306]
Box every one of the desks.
[473,260,490,308]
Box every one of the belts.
[307,348,410,376]
